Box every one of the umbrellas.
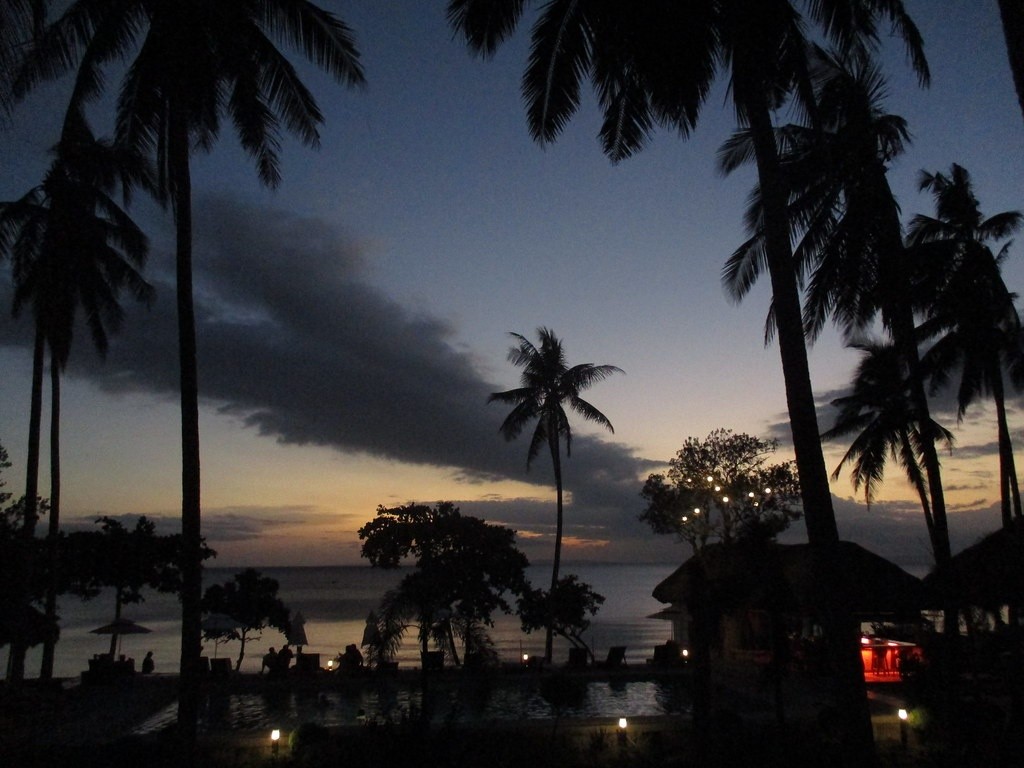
[87,621,153,659]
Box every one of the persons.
[339,645,364,672]
[259,645,303,673]
[92,651,154,674]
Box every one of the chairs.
[299,653,320,669]
[565,647,588,669]
[609,645,628,666]
[647,642,676,667]
[212,658,231,674]
[82,655,136,676]
[422,651,446,671]
